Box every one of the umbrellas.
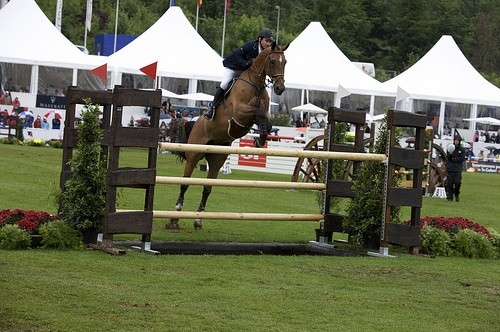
[464,117,500,129]
[140,88,214,116]
[365,112,387,122]
[291,102,328,127]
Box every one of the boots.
[205,85,229,121]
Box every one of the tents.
[0,0,500,138]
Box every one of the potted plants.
[48,98,124,245]
[343,105,403,251]
[315,121,353,244]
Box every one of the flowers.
[0,208,60,235]
[401,215,492,239]
[0,134,63,148]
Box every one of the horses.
[166,39,290,230]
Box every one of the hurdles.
[59,88,428,255]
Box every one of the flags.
[56,0,62,32]
[198,0,202,8]
[85,0,92,31]
[224,0,230,17]
[170,0,175,6]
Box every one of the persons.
[445,136,466,201]
[272,131,281,141]
[294,118,327,129]
[469,129,500,163]
[0,90,60,128]
[161,99,195,119]
[297,134,305,144]
[205,28,275,121]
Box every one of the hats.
[258,28,275,41]
